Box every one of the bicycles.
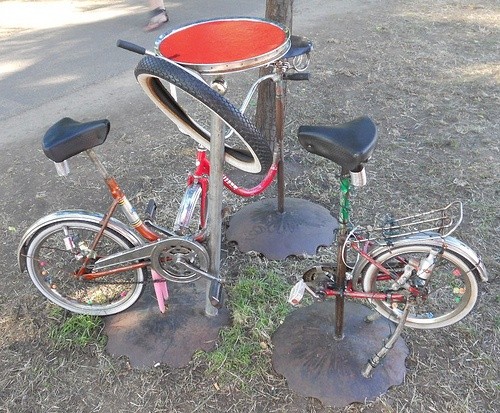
[14,116,227,316]
[115,33,315,240]
[288,113,489,333]
